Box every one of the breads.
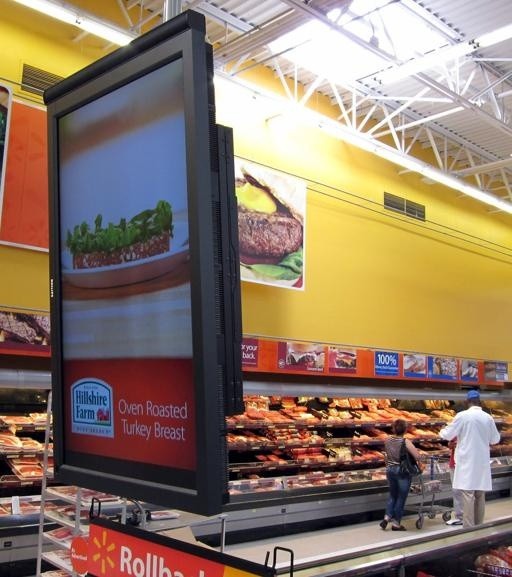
[235,178,303,263]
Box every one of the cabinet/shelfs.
[224,418,512,502]
[35,391,126,577]
[0,399,58,533]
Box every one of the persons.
[446,437,464,524]
[380,420,423,531]
[438,390,501,527]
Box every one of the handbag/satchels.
[399,441,422,479]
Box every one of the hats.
[467,390,480,399]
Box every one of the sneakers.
[380,521,387,529]
[446,518,463,525]
[392,525,405,531]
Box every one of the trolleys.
[411,448,457,530]
